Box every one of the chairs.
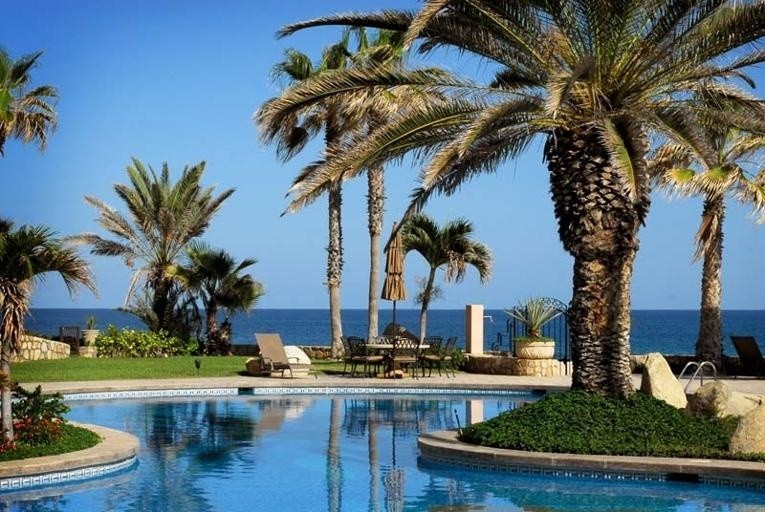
[254,332,318,380]
[730,335,765,380]
[339,335,458,380]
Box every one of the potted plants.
[81,315,99,346]
[504,294,564,360]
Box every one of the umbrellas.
[379,221,406,347]
